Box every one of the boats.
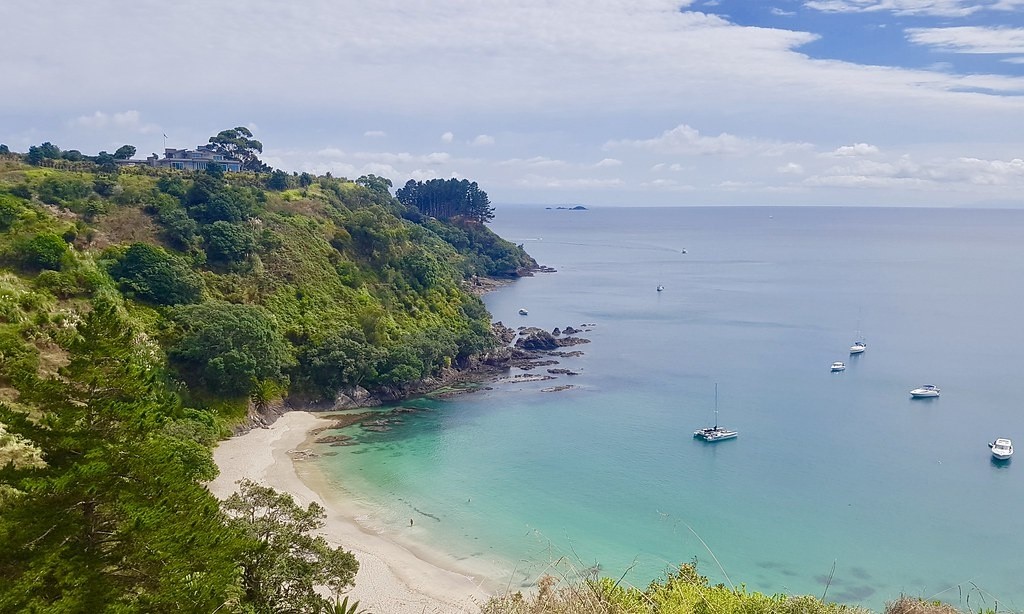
[910,384,941,398]
[849,341,867,355]
[657,285,664,291]
[830,362,846,372]
[987,438,1014,460]
[681,247,687,254]
[519,308,528,315]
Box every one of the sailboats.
[694,382,738,442]
